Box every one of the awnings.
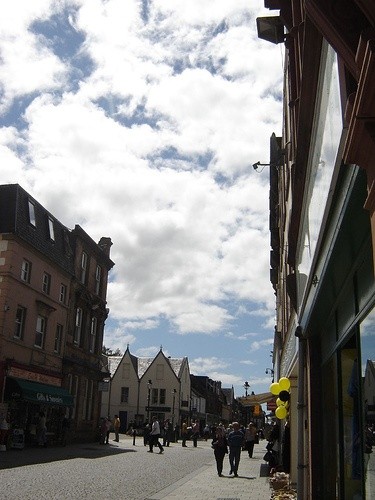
[5,376,74,406]
[239,392,273,406]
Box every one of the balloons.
[270,377,294,419]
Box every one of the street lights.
[172,388,176,424]
[146,379,151,423]
[242,381,251,426]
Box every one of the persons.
[129,416,246,447]
[36,411,47,448]
[101,415,110,445]
[114,414,120,442]
[244,422,258,458]
[211,428,228,477]
[228,422,244,477]
[148,416,164,454]
